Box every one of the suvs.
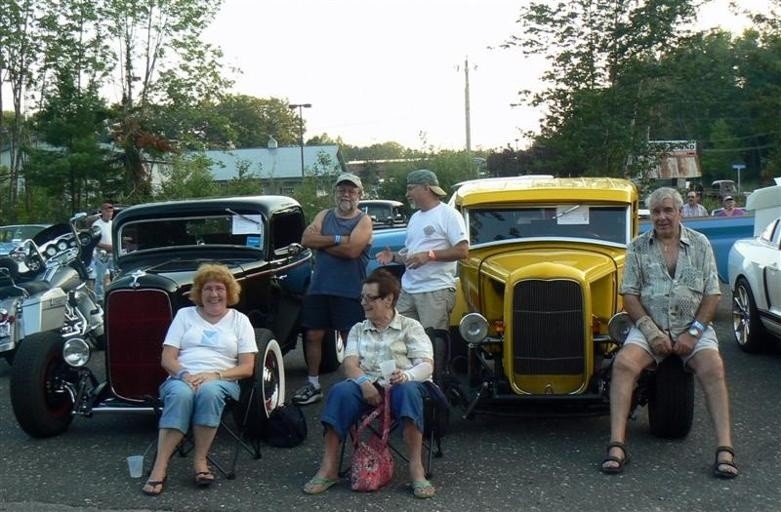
[10,195,346,439]
[406,172,695,441]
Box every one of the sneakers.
[293,381,323,404]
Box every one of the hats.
[335,173,363,190]
[407,169,447,197]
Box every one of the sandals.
[713,446,738,478]
[601,440,631,473]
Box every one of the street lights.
[733,164,746,200]
[287,103,312,184]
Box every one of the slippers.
[142,474,167,495]
[194,470,215,485]
[303,474,340,494]
[411,480,435,498]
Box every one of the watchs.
[400,372,407,381]
[688,328,700,337]
[428,249,436,261]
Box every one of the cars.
[75,206,126,231]
[727,216,780,351]
[362,201,757,286]
[703,179,740,201]
[0,224,56,259]
[354,200,406,231]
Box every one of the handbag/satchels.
[351,436,394,490]
[266,402,307,448]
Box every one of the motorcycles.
[0,211,106,366]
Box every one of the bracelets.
[334,234,342,247]
[175,369,188,381]
[392,251,399,262]
[214,370,223,379]
[355,375,370,386]
[691,320,705,334]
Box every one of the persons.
[301,269,436,498]
[602,187,738,481]
[90,203,119,304]
[142,264,259,497]
[716,194,746,217]
[680,191,709,217]
[293,172,372,408]
[375,168,470,437]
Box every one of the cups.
[379,358,398,386]
[126,454,145,478]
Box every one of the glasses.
[361,293,380,301]
